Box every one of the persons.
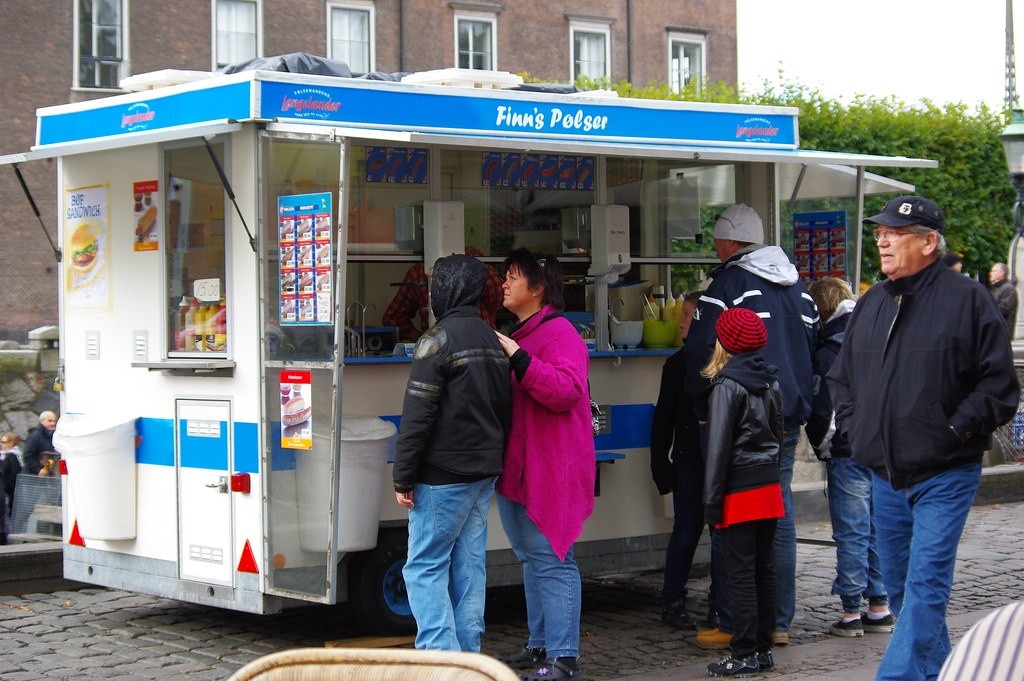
[649,289,737,633]
[699,307,786,678]
[804,276,896,636]
[693,201,821,650]
[493,247,597,681]
[943,251,1019,341]
[824,195,1022,681]
[392,253,514,652]
[0,410,62,546]
[381,222,505,343]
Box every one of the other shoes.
[772,632,788,644]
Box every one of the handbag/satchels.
[587,378,602,437]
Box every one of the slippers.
[494,642,547,669]
[522,656,581,681]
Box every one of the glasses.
[873,230,920,241]
[534,252,547,278]
[1,440,11,444]
[714,213,736,230]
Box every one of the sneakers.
[861,613,895,633]
[831,617,864,638]
[706,650,775,678]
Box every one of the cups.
[652,285,665,322]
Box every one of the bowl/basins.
[612,321,645,349]
[643,320,676,348]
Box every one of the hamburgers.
[70,224,99,272]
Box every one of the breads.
[795,230,846,271]
[281,396,312,426]
[281,218,330,316]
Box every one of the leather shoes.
[662,605,698,630]
[695,628,733,649]
[707,605,720,628]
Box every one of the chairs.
[225,646,524,681]
[931,598,1024,681]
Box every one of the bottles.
[642,293,660,320]
[666,291,685,347]
[172,295,227,352]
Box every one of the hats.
[862,196,945,235]
[944,254,961,267]
[713,203,765,245]
[715,308,768,354]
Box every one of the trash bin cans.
[52,413,137,541]
[295,415,397,553]
